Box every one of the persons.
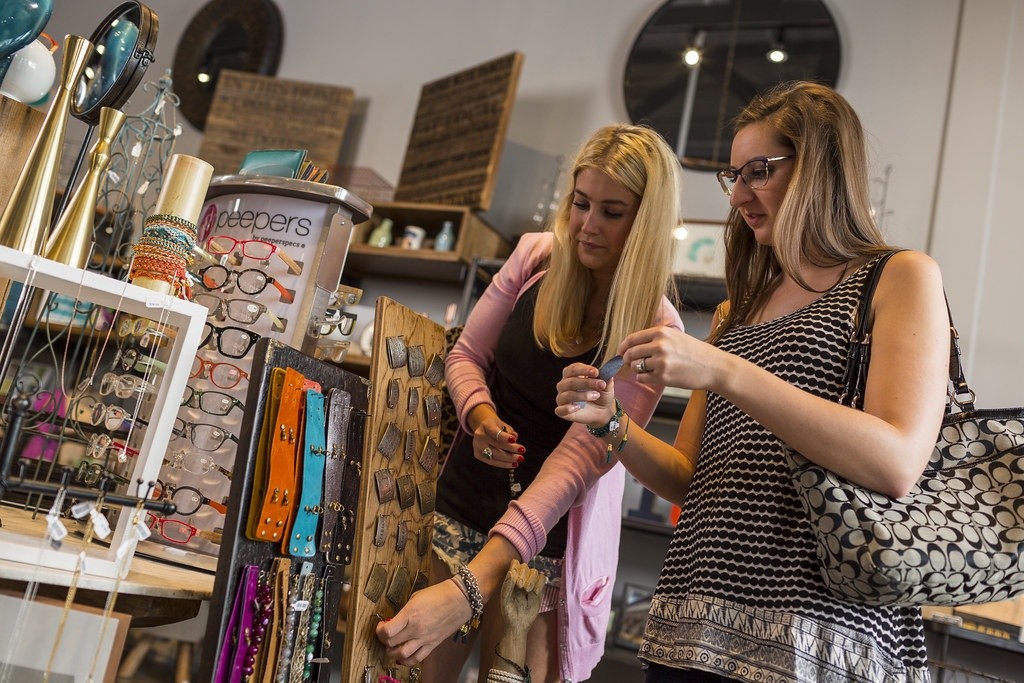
[553,80,951,683]
[377,122,685,683]
[487,559,548,683]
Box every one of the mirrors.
[621,0,843,173]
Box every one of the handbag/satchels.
[434,252,551,467]
[781,249,1024,606]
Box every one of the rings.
[496,431,501,440]
[637,357,649,373]
[480,445,495,460]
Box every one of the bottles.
[434,221,454,252]
[366,218,394,248]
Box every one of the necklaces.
[117,269,180,463]
[0,483,146,683]
[62,242,136,401]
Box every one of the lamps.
[765,25,786,63]
[682,28,708,68]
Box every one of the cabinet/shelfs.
[347,200,510,284]
[589,275,730,683]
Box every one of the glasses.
[44,235,357,549]
[716,156,797,196]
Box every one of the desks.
[0,505,217,683]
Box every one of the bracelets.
[451,565,484,644]
[487,642,533,683]
[587,398,629,464]
[128,214,197,299]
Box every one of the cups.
[399,225,424,250]
